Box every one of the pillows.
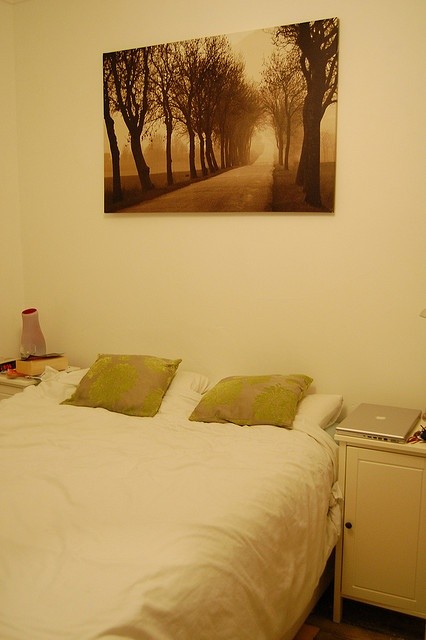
[189,371,314,430]
[58,353,183,417]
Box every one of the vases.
[20,307,46,356]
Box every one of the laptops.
[335,402,422,444]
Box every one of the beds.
[0,367,344,640]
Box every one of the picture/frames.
[102,17,341,214]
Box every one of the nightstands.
[0,366,81,402]
[333,420,426,624]
